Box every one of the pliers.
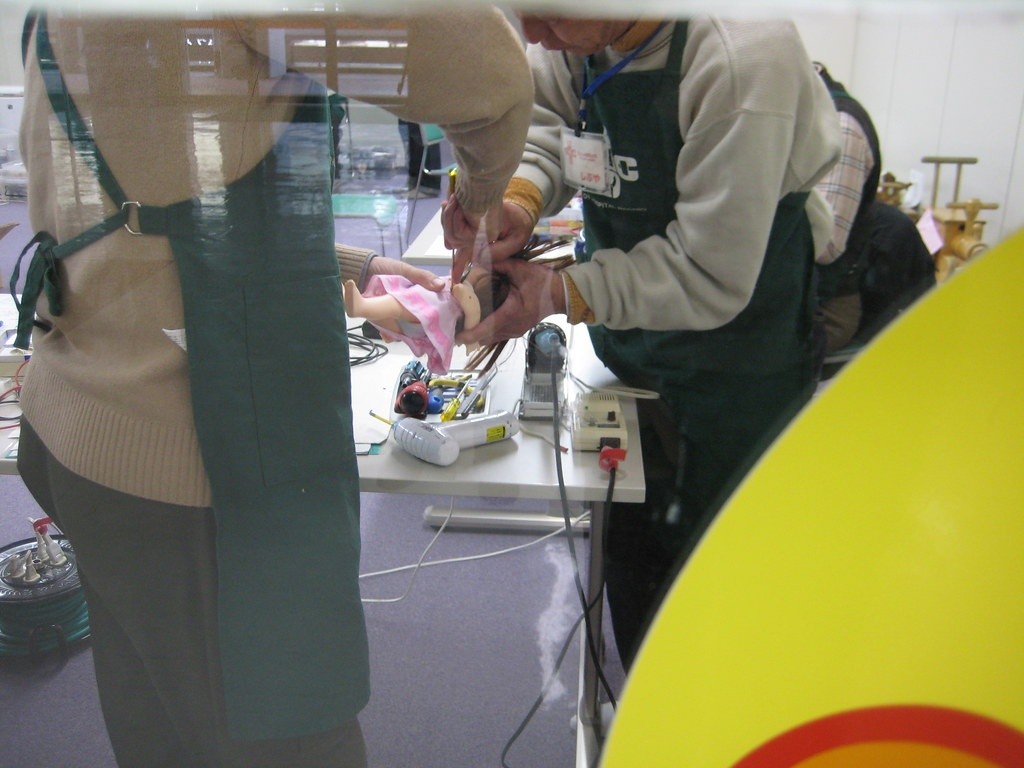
[429,373,483,409]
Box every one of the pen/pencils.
[461,367,498,416]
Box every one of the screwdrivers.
[439,378,470,422]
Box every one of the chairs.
[325,92,450,254]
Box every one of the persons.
[452,0,844,737]
[10,0,535,768]
[813,60,883,356]
[859,202,938,345]
[344,234,577,376]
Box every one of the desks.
[0,291,646,768]
[400,194,591,536]
